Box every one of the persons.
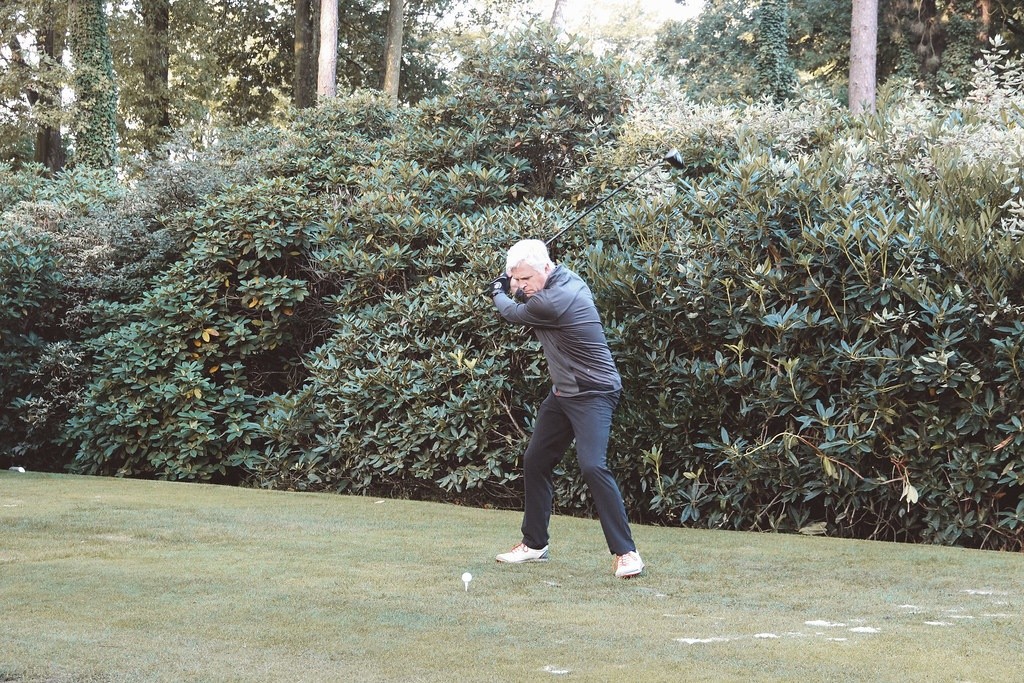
[489,239,644,578]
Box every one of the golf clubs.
[546,147,687,246]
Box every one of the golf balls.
[462,572,472,582]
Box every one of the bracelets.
[489,291,504,299]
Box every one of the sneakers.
[615,550,645,578]
[496,544,549,563]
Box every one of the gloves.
[490,274,511,298]
